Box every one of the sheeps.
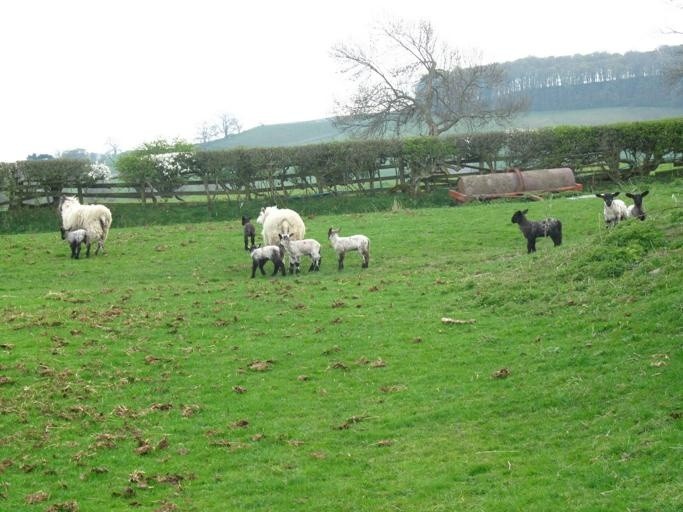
[510,208,563,254]
[255,204,306,245]
[246,242,286,279]
[595,191,628,225]
[241,214,257,249]
[58,193,113,257]
[326,227,370,271]
[624,190,650,222]
[60,225,90,258]
[278,232,321,274]
